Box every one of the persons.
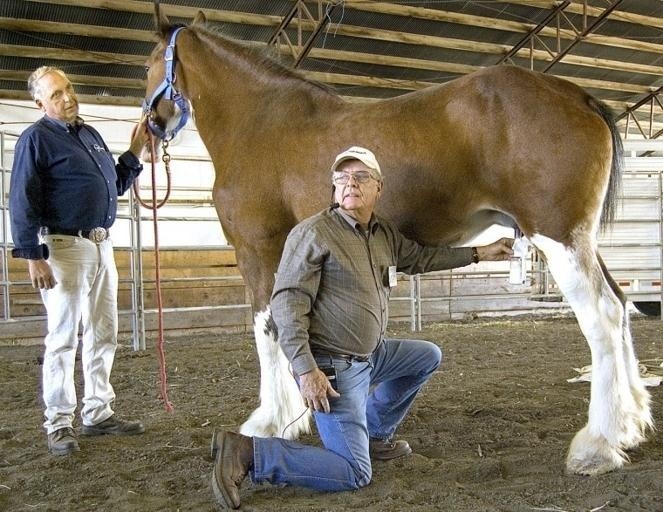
[8,65,151,456]
[211,145,520,509]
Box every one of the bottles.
[507,227,527,286]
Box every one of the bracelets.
[472,247,479,264]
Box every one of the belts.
[41,227,108,242]
[309,348,369,361]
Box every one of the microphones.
[331,184,339,210]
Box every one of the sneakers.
[82,415,145,436]
[370,438,412,460]
[47,428,81,455]
[210,428,253,509]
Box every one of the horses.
[138,1,654,478]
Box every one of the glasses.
[335,171,379,184]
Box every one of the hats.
[330,146,381,174]
